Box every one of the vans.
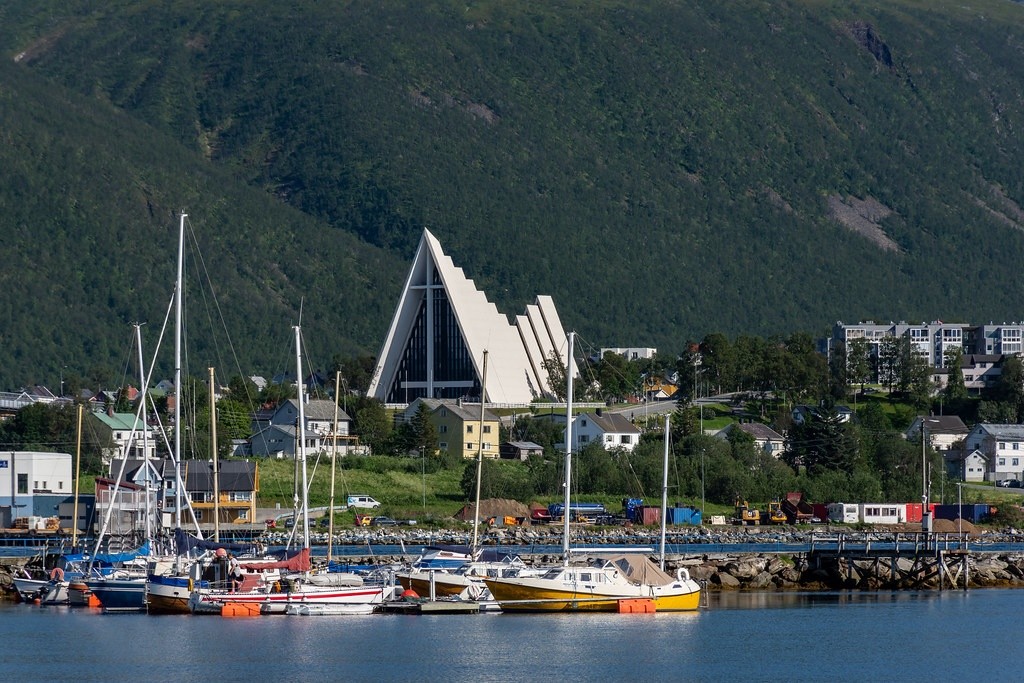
[346,495,381,509]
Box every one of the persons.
[227,553,242,595]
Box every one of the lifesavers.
[677,568,690,580]
[51,567,64,580]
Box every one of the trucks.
[781,492,814,525]
[826,503,859,524]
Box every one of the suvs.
[356,515,366,526]
[320,519,330,529]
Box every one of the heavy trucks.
[547,497,643,527]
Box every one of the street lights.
[826,336,831,388]
[957,483,961,548]
[419,446,426,507]
[938,471,947,504]
[920,419,940,513]
[641,376,645,402]
[60,365,68,396]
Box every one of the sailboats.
[11,205,701,615]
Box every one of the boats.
[973,541,994,546]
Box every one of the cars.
[265,519,276,529]
[306,517,316,527]
[996,479,1022,488]
[285,518,295,528]
[370,516,400,528]
[361,517,375,526]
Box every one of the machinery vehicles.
[759,502,787,526]
[731,493,760,526]
